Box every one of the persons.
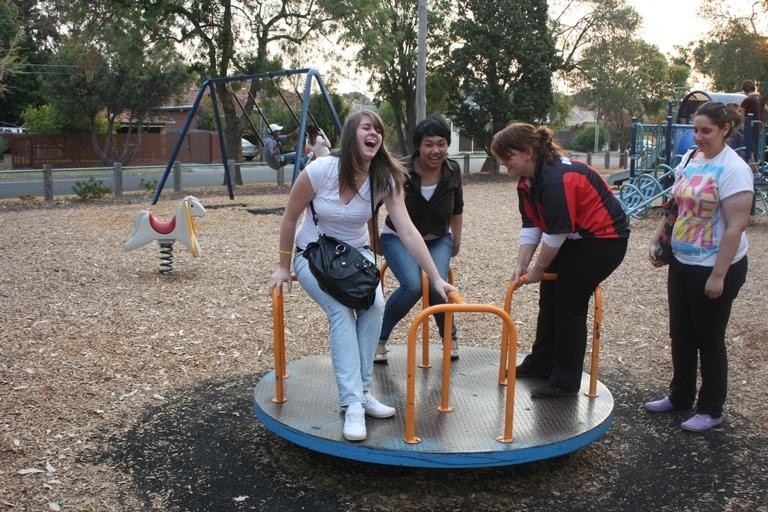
[264,123,306,170]
[268,109,459,443]
[726,81,768,180]
[491,122,629,398]
[303,122,331,157]
[644,101,754,434]
[363,114,462,361]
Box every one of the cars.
[241,137,259,162]
[628,140,656,156]
[0,126,30,134]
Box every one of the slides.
[606,138,673,186]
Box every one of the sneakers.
[306,151,314,164]
[681,414,723,432]
[342,403,367,441]
[362,393,396,419]
[643,396,674,413]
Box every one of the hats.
[268,123,284,134]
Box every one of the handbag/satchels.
[651,148,698,266]
[302,233,380,311]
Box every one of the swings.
[225,78,287,170]
[270,75,332,157]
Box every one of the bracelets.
[535,260,549,269]
[278,250,293,256]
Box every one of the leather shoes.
[530,381,579,398]
[506,356,541,377]
[373,349,387,363]
[450,347,459,358]
